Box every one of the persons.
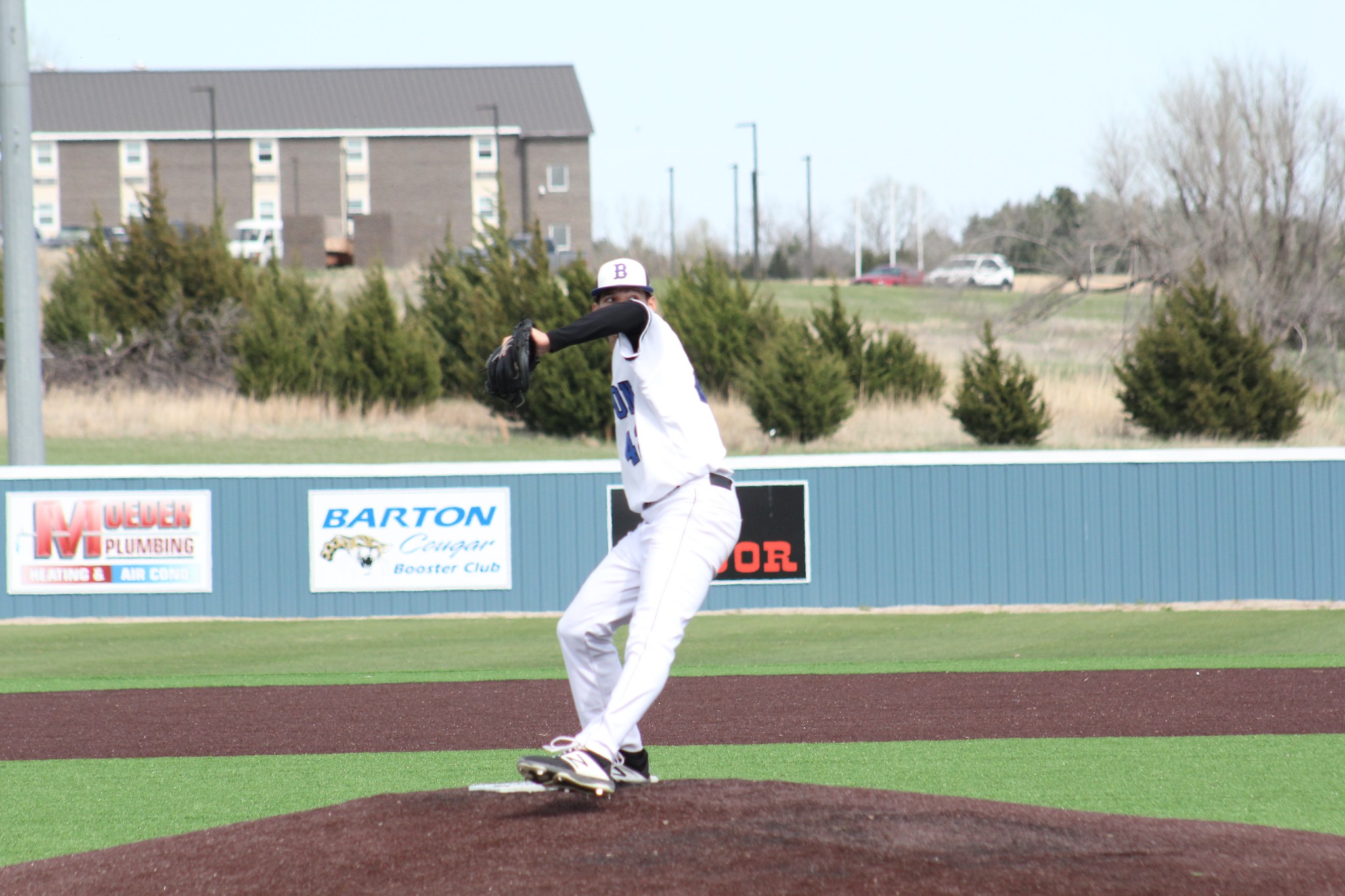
[485,257,742,795]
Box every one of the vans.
[227,219,285,267]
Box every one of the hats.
[593,259,654,296]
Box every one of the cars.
[34,220,206,251]
[850,265,924,287]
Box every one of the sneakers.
[518,734,615,802]
[615,750,650,784]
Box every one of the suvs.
[923,253,1015,293]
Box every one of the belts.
[710,473,733,490]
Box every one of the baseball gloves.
[479,319,542,412]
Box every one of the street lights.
[475,103,503,231]
[731,162,739,268]
[667,164,675,267]
[804,155,812,278]
[192,84,221,235]
[738,119,759,280]
